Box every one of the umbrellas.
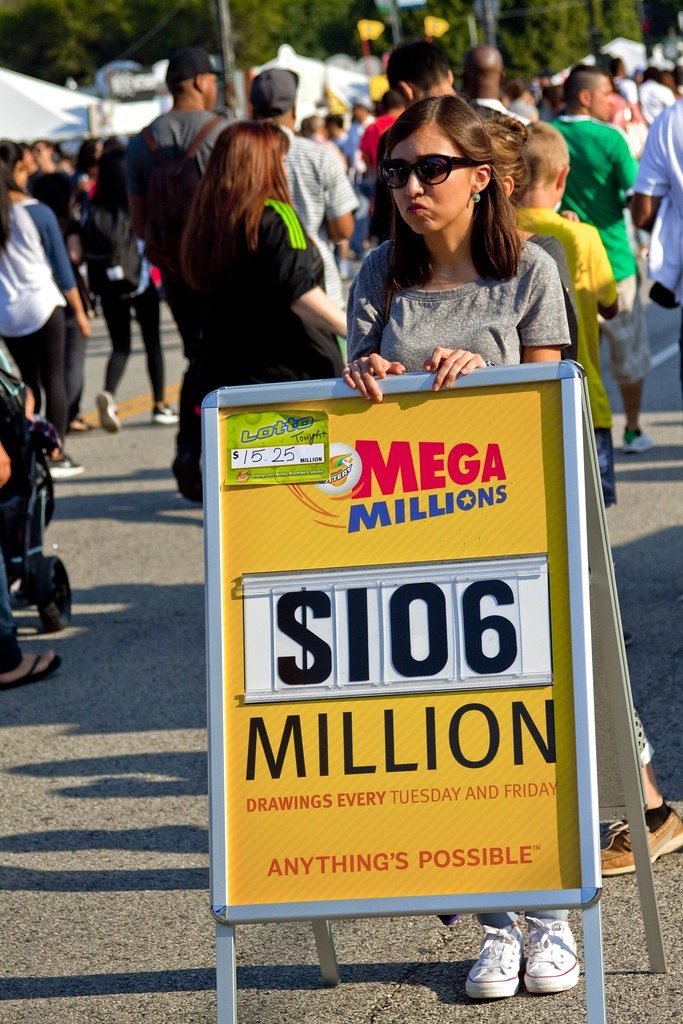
[245,42,376,130]
[550,36,676,86]
[0,65,147,145]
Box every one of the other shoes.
[46,458,83,478]
[68,417,95,432]
[96,394,119,433]
[152,406,181,424]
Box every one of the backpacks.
[142,112,227,280]
[80,202,142,297]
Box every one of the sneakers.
[465,914,522,1001]
[524,915,578,993]
[623,425,653,453]
[600,808,683,875]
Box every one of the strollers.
[0,348,73,631]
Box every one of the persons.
[370,38,683,874]
[121,46,238,338]
[166,120,350,391]
[0,135,181,688]
[298,91,408,283]
[338,94,579,1000]
[247,67,359,313]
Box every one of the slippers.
[0,656,62,692]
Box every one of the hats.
[166,52,224,77]
[250,68,297,116]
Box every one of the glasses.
[380,154,486,188]
